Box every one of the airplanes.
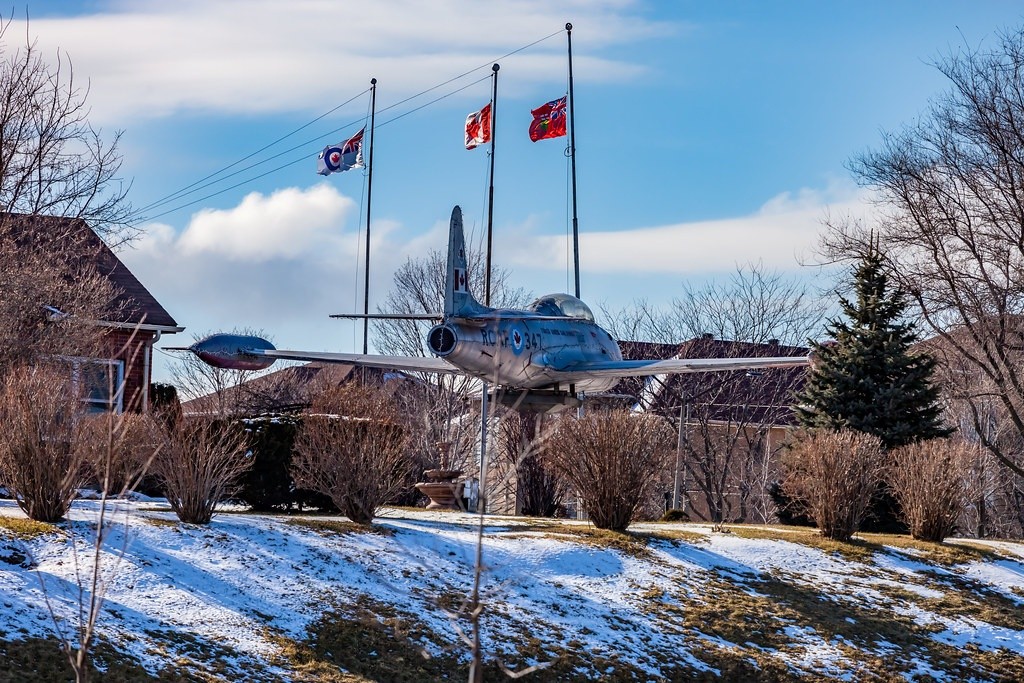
[141,196,815,426]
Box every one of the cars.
[220,412,382,521]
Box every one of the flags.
[317,127,366,176]
[529,95,567,143]
[464,100,492,150]
